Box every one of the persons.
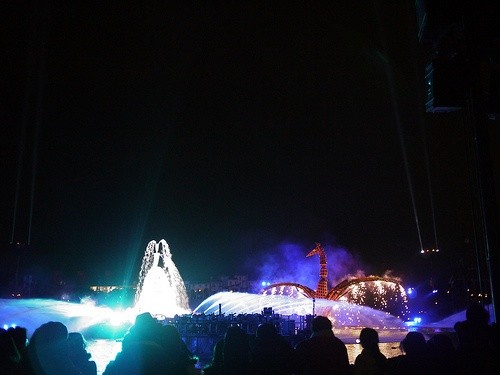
[390,304,500,375]
[352,327,389,375]
[253,323,295,375]
[0,315,198,375]
[295,317,351,375]
[222,326,253,375]
